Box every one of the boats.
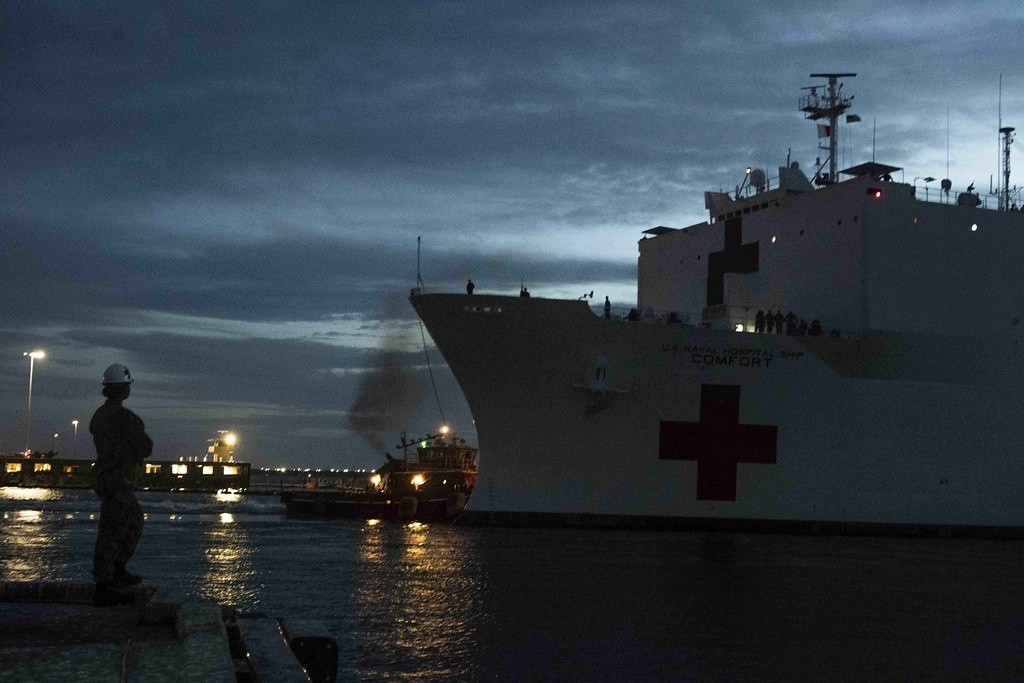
[1,457,251,489]
[280,482,399,521]
[409,71,1024,544]
[385,430,479,497]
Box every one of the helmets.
[102,364,134,384]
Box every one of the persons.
[755,309,820,337]
[521,288,529,297]
[467,279,475,294]
[90,363,153,585]
[604,296,610,318]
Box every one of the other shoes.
[110,572,143,589]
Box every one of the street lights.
[72,421,79,457]
[26,352,45,449]
